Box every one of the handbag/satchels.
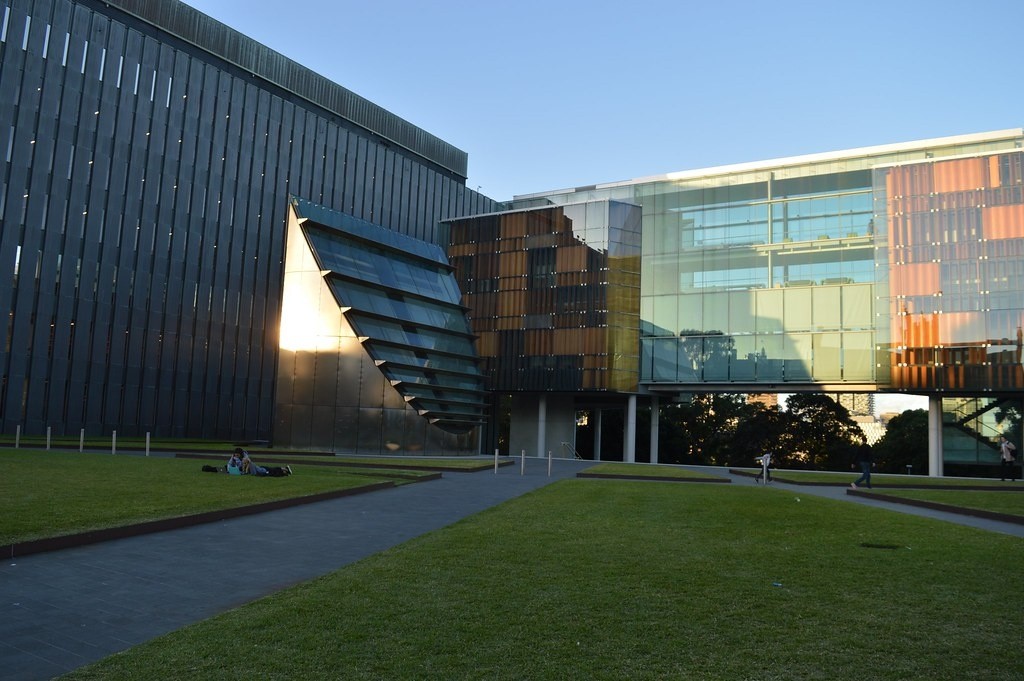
[1007,442,1018,458]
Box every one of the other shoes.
[285,465,292,475]
[851,483,857,489]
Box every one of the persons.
[227,448,248,475]
[851,437,875,490]
[242,457,292,477]
[1000,436,1017,481]
[754,450,773,483]
